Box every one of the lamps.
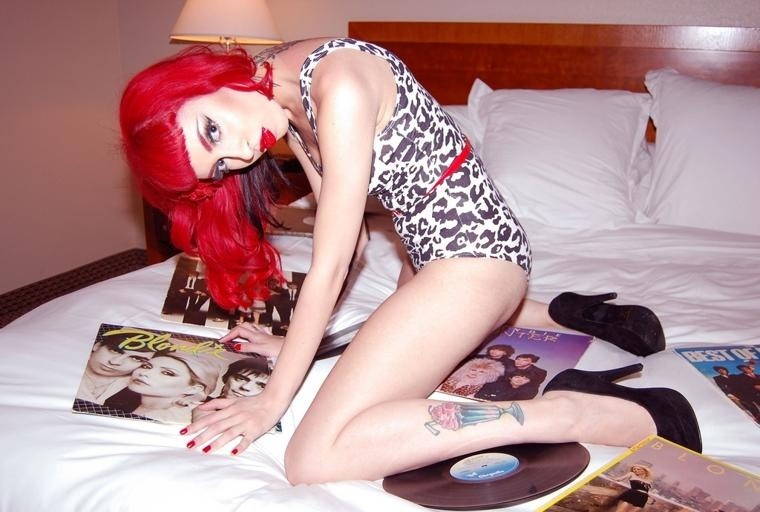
[168,1,284,55]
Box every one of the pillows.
[634,67,760,236]
[465,76,659,234]
[285,105,464,216]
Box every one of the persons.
[173,268,298,330]
[611,465,652,512]
[712,365,760,424]
[75,332,272,426]
[118,37,703,485]
[436,344,547,401]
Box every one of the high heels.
[542,363,702,454]
[548,292,665,357]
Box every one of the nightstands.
[142,162,313,269]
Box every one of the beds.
[0,23,760,512]
